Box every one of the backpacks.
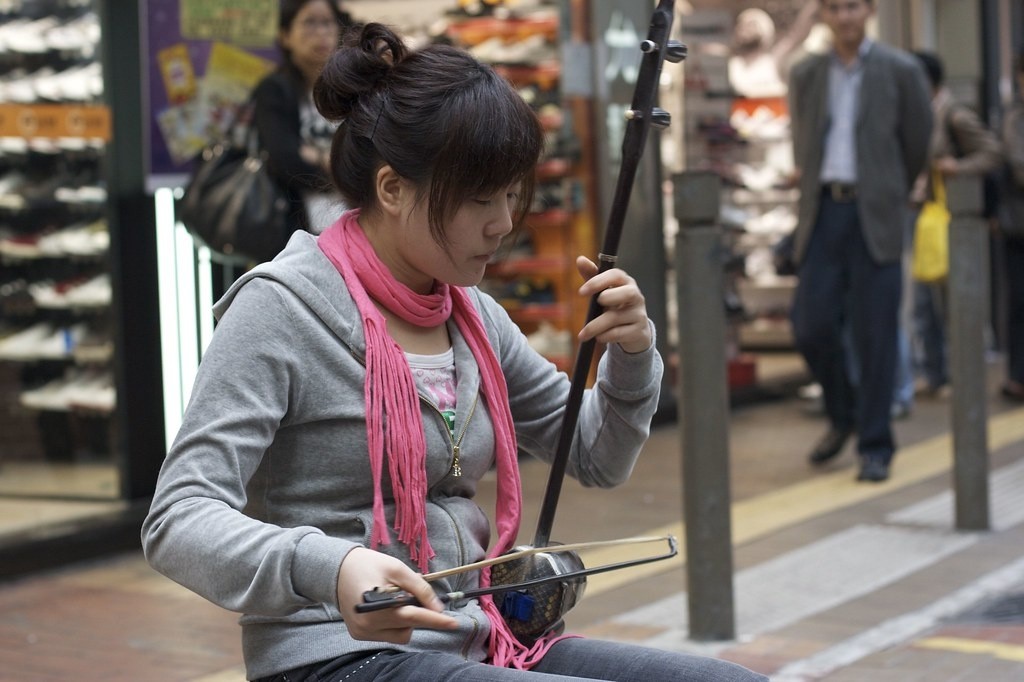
[944,102,1010,220]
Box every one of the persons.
[140,22,768,682]
[229,0,366,282]
[788,0,1024,482]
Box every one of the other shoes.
[480,74,582,360]
[395,0,562,70]
[700,56,802,324]
[854,456,890,483]
[0,0,117,418]
[809,425,853,462]
[909,378,955,400]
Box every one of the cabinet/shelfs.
[448,0,606,389]
[735,98,803,353]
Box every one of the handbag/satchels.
[177,72,300,254]
[911,169,952,280]
